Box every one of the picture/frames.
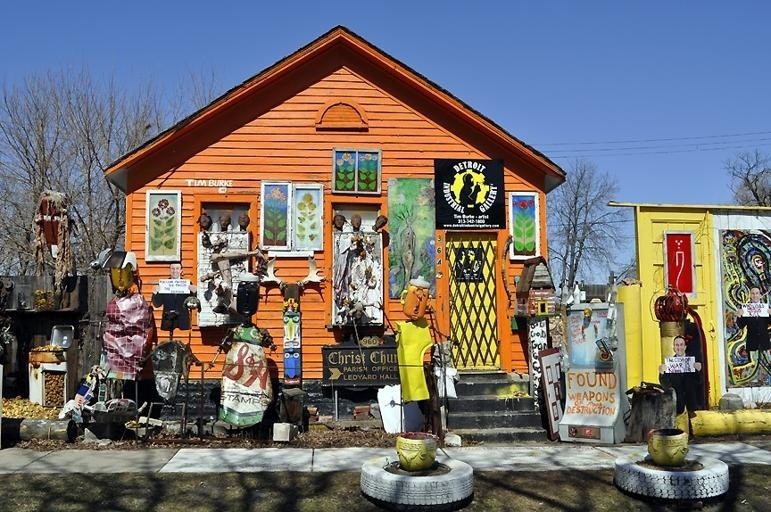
[260,181,295,252]
[292,183,326,252]
[144,188,184,263]
[508,190,543,262]
[331,232,385,326]
[662,230,698,297]
[331,146,384,195]
[196,230,253,327]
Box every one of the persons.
[659,337,702,412]
[736,286,771,352]
[152,263,197,330]
[456,250,481,279]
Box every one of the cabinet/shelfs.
[29,348,78,409]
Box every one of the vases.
[396,430,441,472]
[647,427,691,464]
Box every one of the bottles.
[574,280,587,305]
[561,278,569,303]
[605,274,617,303]
